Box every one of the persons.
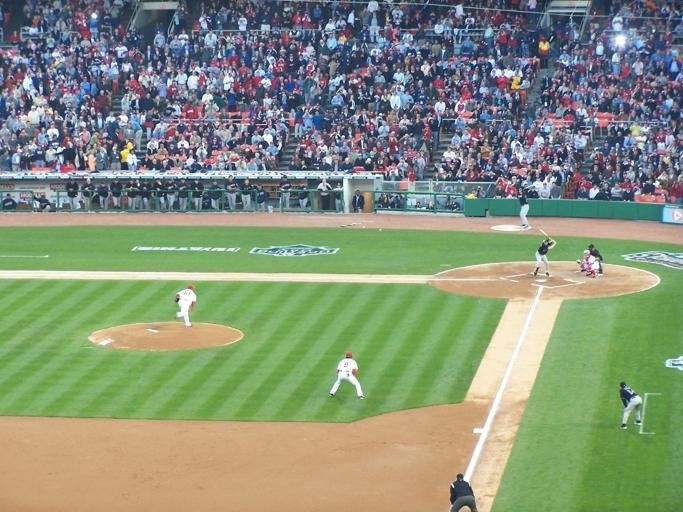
[517,187,529,229]
[620,382,642,430]
[533,237,556,276]
[328,351,365,400]
[175,284,196,328]
[576,244,602,278]
[449,473,478,512]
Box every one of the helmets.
[346,352,352,357]
[187,284,192,288]
[457,473,463,481]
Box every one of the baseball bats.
[539,228,556,244]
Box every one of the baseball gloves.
[576,259,581,264]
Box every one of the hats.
[620,382,626,388]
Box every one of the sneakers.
[619,424,627,430]
[634,420,642,425]
